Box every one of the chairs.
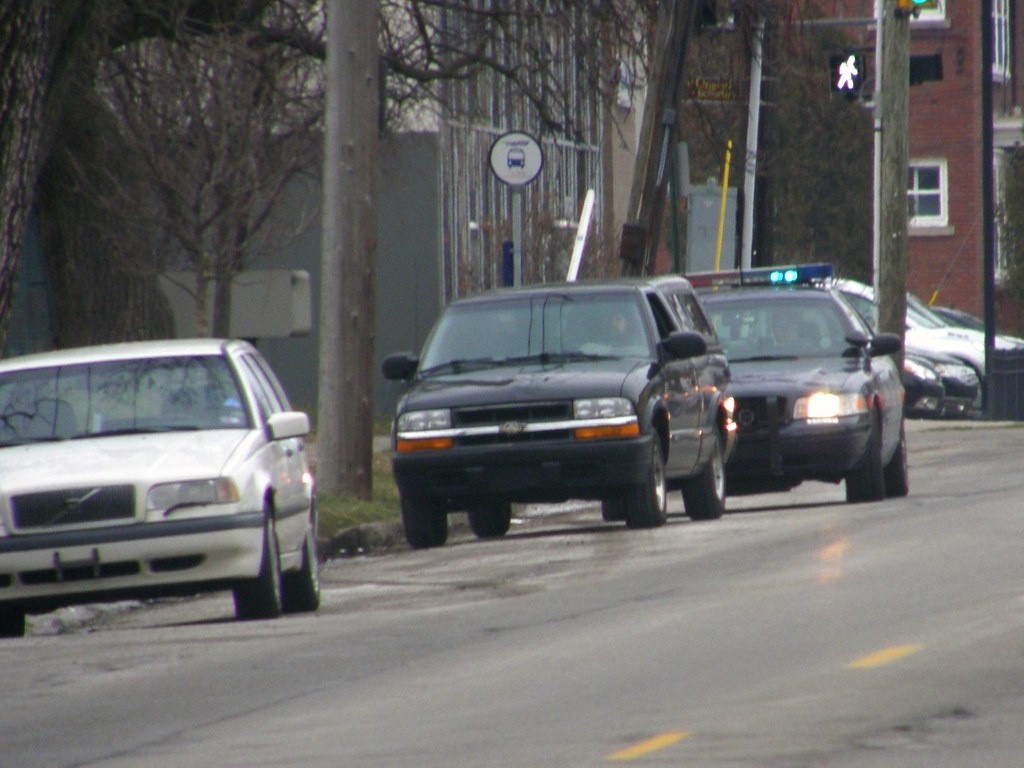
[20,398,77,438]
[161,385,221,425]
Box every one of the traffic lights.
[830,55,864,92]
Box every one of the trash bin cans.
[992,348,1024,419]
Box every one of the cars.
[832,277,1024,419]
[0,337,319,640]
[690,262,906,504]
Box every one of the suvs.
[384,274,736,548]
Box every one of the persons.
[771,306,803,348]
[608,309,636,346]
